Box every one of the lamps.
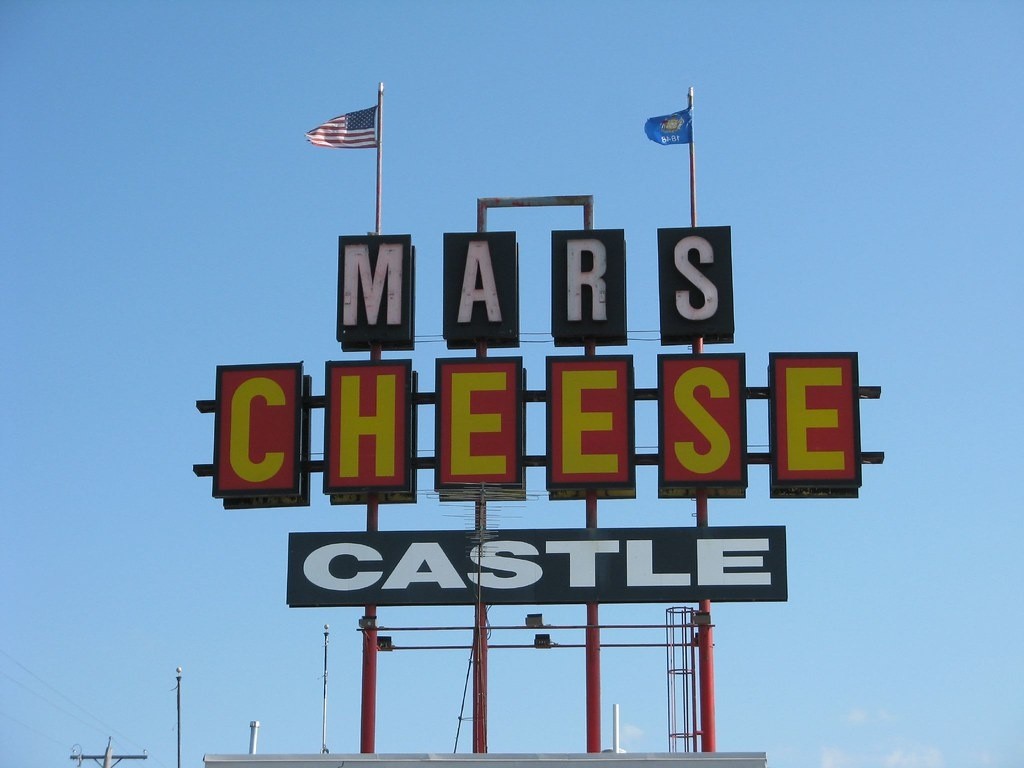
[377,636,395,651]
[534,634,550,649]
[525,614,544,625]
[359,616,378,628]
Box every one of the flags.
[644,107,694,145]
[304,105,378,149]
